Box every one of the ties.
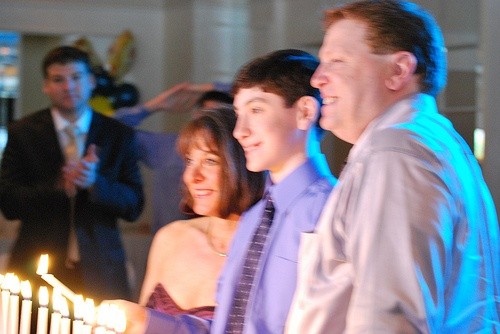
[223,195,274,334]
[65,125,81,263]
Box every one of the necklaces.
[206,219,228,257]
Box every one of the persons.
[99,49,340,333]
[0,46,146,305]
[113,81,235,236]
[138,106,266,321]
[281,0,500,334]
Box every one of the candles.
[0,253,127,334]
